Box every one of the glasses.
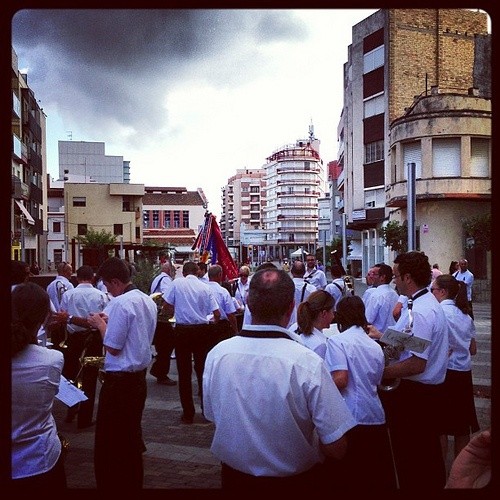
[239,274,248,277]
[392,274,401,283]
[370,273,379,278]
[431,287,448,293]
[63,262,66,269]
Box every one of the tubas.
[149,253,177,324]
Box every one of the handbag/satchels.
[150,276,174,321]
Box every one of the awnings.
[15,199,36,225]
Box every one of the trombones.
[341,275,356,299]
[55,280,70,349]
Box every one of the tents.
[291,249,310,264]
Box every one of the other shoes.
[150,368,169,381]
[180,415,192,424]
[156,376,177,386]
[78,421,97,428]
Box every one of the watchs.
[67,315,73,325]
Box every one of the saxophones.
[377,294,415,392]
[78,330,106,385]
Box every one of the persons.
[11,252,491,489]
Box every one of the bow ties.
[408,289,428,310]
